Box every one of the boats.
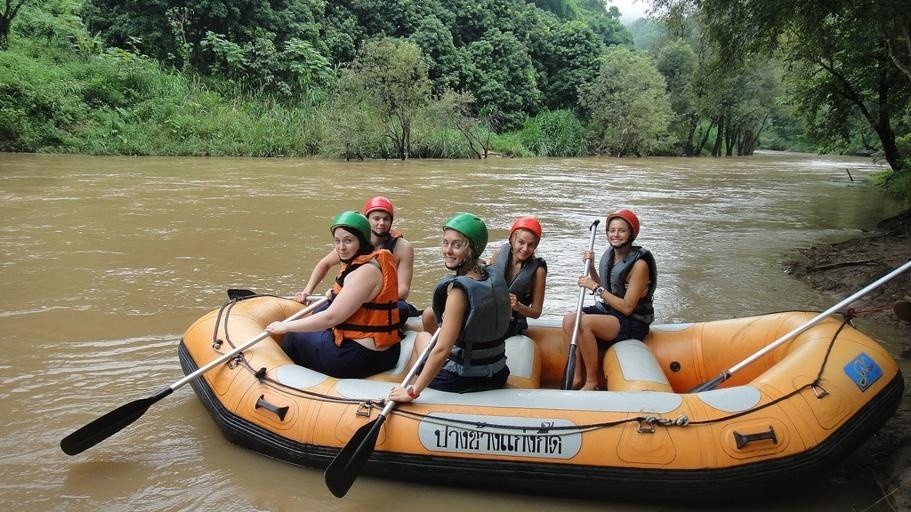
[177,293,906,503]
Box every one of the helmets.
[363,196,393,218]
[330,211,371,240]
[509,218,541,249]
[606,209,639,239]
[443,213,487,259]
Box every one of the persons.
[561,209,659,390]
[293,195,414,328]
[388,211,514,403]
[419,215,549,339]
[264,209,404,380]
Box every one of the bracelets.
[593,284,602,292]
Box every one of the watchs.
[597,286,606,295]
[407,386,421,399]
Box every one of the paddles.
[562,220,602,390]
[226,288,332,303]
[324,324,440,497]
[687,261,911,395]
[60,295,339,456]
